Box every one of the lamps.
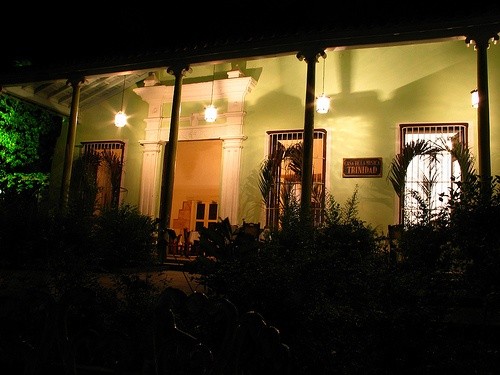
[315,54,330,113]
[204,64,218,122]
[113,74,129,128]
